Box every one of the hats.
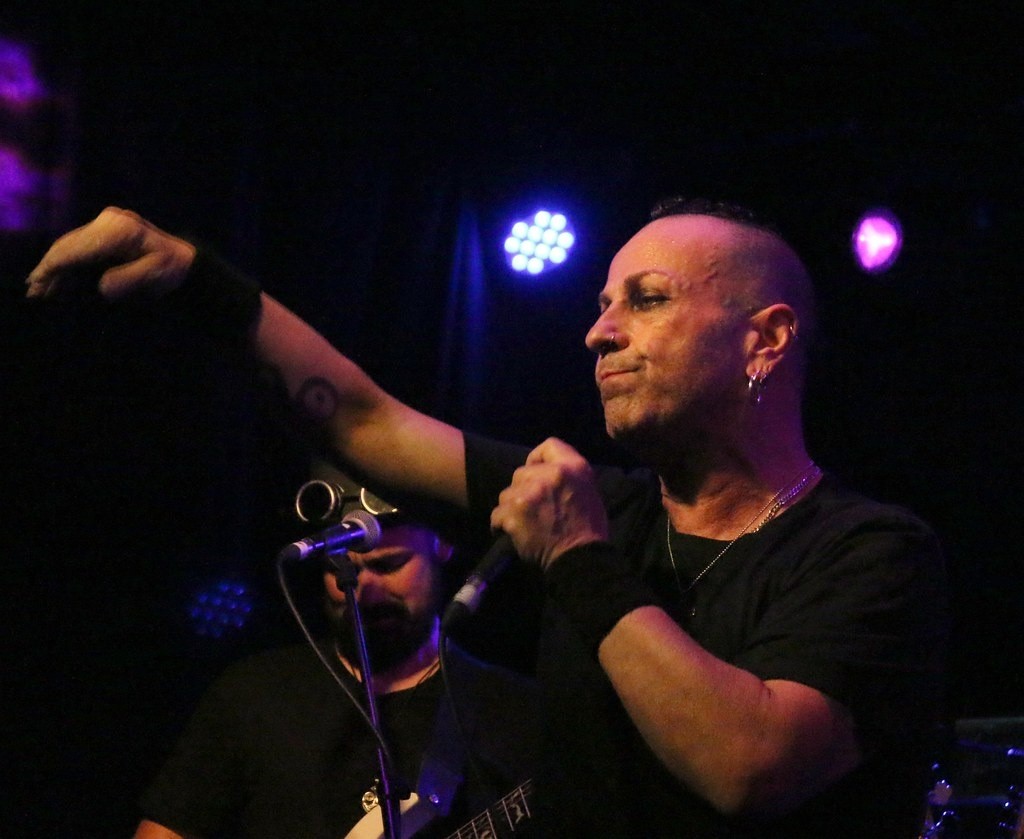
[265,448,416,529]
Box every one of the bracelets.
[544,539,663,657]
[163,245,262,350]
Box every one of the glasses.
[295,479,401,524]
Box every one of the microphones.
[278,511,383,564]
[439,533,519,634]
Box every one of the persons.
[27,195,942,839]
[132,461,456,839]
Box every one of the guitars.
[342,776,562,839]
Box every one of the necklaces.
[348,660,439,729]
[667,461,822,616]
